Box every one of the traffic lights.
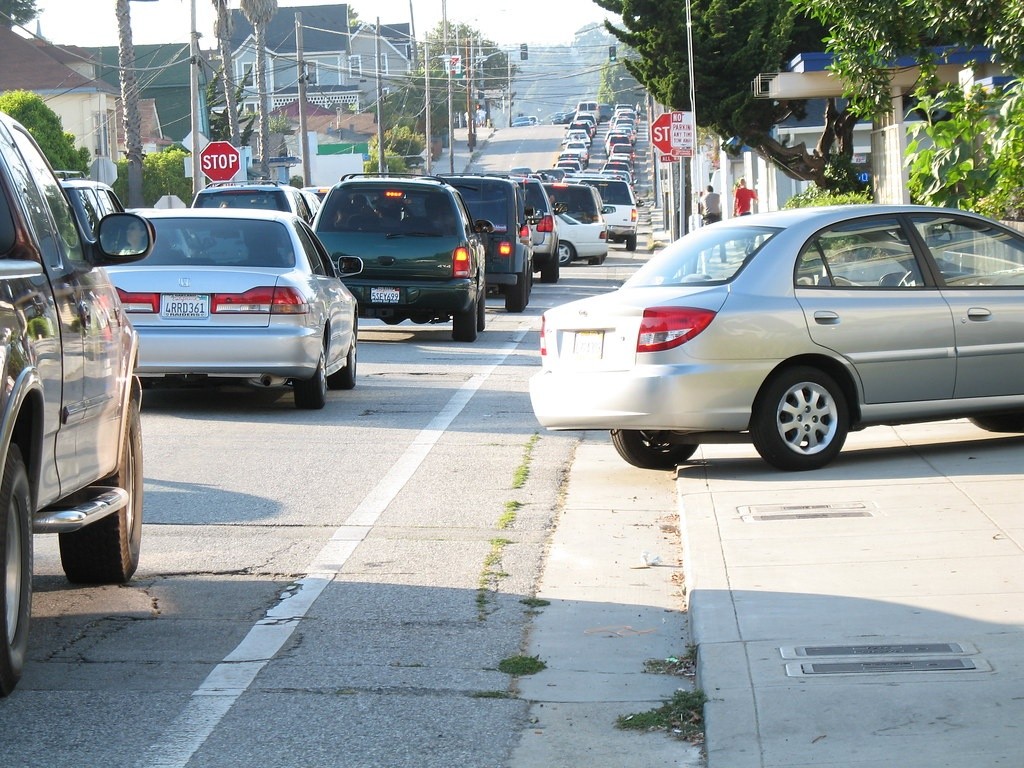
[608,46,616,62]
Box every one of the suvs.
[185,179,347,231]
[487,172,569,284]
[307,170,496,343]
[405,171,535,313]
[0,108,157,699]
[53,167,126,267]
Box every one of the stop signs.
[198,140,241,183]
[650,112,672,154]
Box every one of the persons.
[636,102,642,122]
[463,105,488,128]
[114,218,148,256]
[732,177,759,217]
[701,185,722,226]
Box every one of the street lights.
[468,33,489,147]
[423,31,451,177]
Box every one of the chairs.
[347,213,374,226]
[400,217,431,230]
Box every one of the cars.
[101,205,366,410]
[499,97,638,268]
[527,201,1024,472]
